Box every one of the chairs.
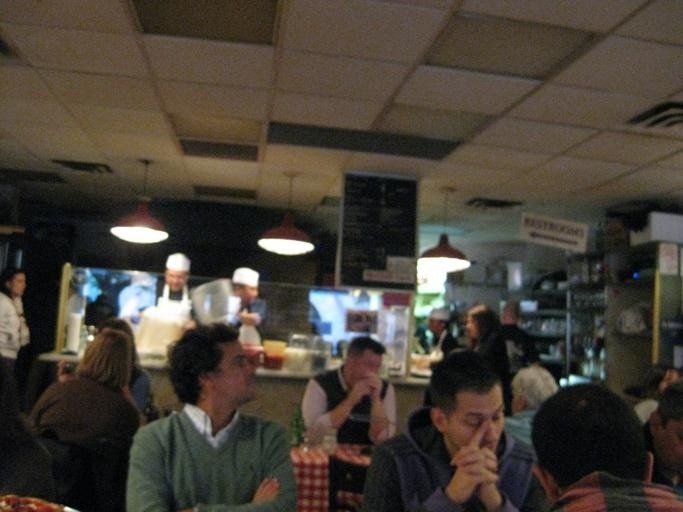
[328,452,371,505]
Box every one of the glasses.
[215,355,252,368]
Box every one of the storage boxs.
[629,211,682,245]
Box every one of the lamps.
[257,171,315,255]
[109,159,169,244]
[416,188,470,274]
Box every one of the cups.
[289,334,309,348]
[311,336,332,371]
[82,325,95,343]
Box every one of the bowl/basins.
[263,340,287,370]
[286,347,308,370]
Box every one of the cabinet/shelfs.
[566,252,605,379]
[607,271,680,410]
[514,307,565,386]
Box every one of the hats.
[232,267,259,289]
[622,364,683,401]
[166,253,191,273]
[431,308,448,321]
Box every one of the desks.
[38,352,431,452]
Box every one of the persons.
[302,337,397,445]
[126,320,298,511]
[500,300,540,365]
[643,381,683,488]
[412,309,460,368]
[59,320,150,411]
[231,268,267,346]
[511,344,560,415]
[86,295,113,329]
[0,266,30,430]
[467,305,505,356]
[362,352,551,512]
[0,435,83,511]
[140,251,198,331]
[29,329,141,448]
[637,363,679,395]
[529,383,683,512]
